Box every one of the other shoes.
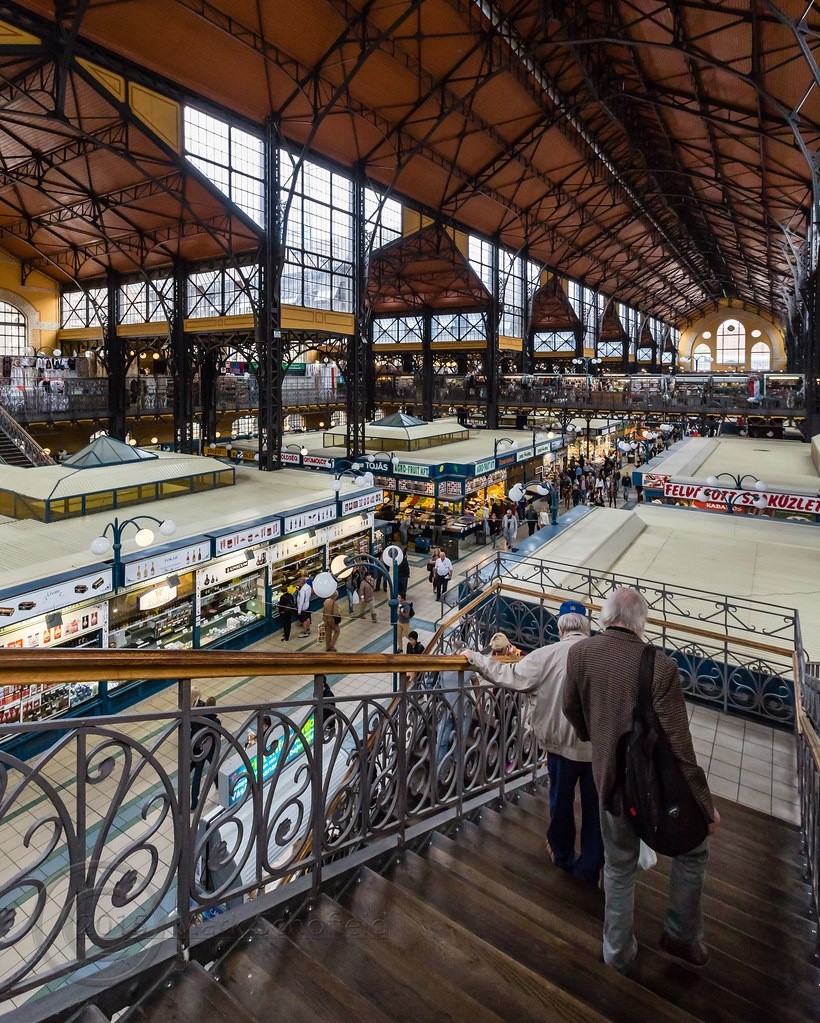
[298,630,311,638]
[281,633,289,641]
[326,647,337,652]
[545,838,558,864]
[657,930,711,971]
[398,649,403,653]
[436,599,440,601]
[433,589,436,593]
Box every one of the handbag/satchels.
[332,601,341,624]
[316,621,326,643]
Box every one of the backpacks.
[400,602,415,618]
[281,593,294,615]
[605,644,710,858]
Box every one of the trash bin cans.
[442,538,459,560]
[415,537,431,554]
[476,531,486,545]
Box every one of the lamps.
[680,355,713,373]
[697,472,768,515]
[618,440,649,465]
[368,451,400,479]
[325,458,375,502]
[642,423,676,450]
[312,545,404,625]
[572,357,602,376]
[23,345,62,359]
[507,480,559,525]
[280,443,309,467]
[208,440,232,458]
[546,423,582,439]
[494,437,519,459]
[90,515,176,596]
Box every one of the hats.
[559,600,586,616]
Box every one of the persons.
[562,589,721,973]
[190,417,743,812]
[459,614,606,886]
